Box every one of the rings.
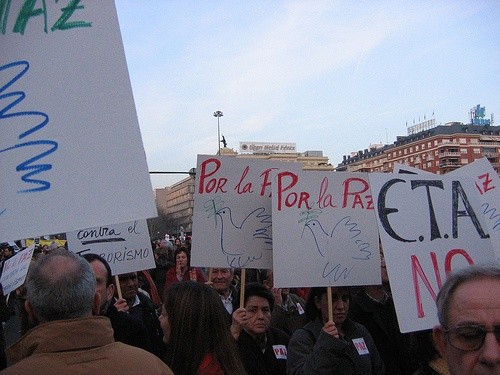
[243,317,246,320]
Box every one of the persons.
[434,264,500,375]
[0,249,174,375]
[0,235,435,375]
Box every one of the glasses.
[440,324,500,352]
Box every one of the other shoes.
[2,322,6,325]
[10,311,16,316]
